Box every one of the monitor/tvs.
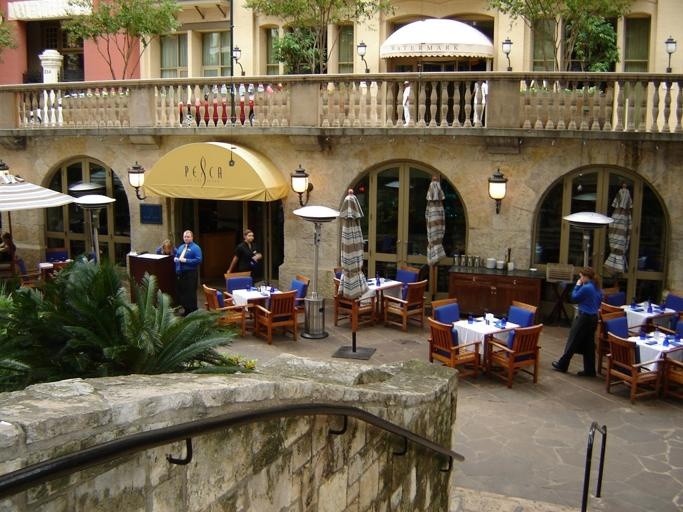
[140,203,162,225]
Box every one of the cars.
[24,91,126,130]
[178,83,283,128]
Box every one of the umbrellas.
[423,175,447,302]
[337,189,370,351]
[602,181,633,289]
[0,172,75,241]
[380,18,495,62]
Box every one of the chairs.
[202,271,310,345]
[0,249,72,289]
[333,265,427,332]
[597,287,682,404]
[427,298,544,389]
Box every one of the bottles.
[626,295,680,347]
[244,279,276,292]
[464,307,509,329]
[374,272,385,286]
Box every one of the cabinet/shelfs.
[448,272,540,323]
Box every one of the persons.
[0,233,18,265]
[173,230,203,315]
[227,229,263,286]
[552,265,602,378]
[402,81,410,127]
[157,240,177,255]
[471,81,490,127]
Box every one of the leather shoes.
[577,371,596,377]
[552,361,567,373]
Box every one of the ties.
[179,244,188,260]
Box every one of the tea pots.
[453,255,480,268]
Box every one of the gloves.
[180,257,186,263]
[174,257,179,263]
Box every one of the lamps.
[357,40,370,73]
[488,167,509,214]
[128,161,147,202]
[0,159,9,176]
[664,33,676,73]
[291,164,313,207]
[502,36,513,71]
[231,44,245,76]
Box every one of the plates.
[486,258,506,270]
